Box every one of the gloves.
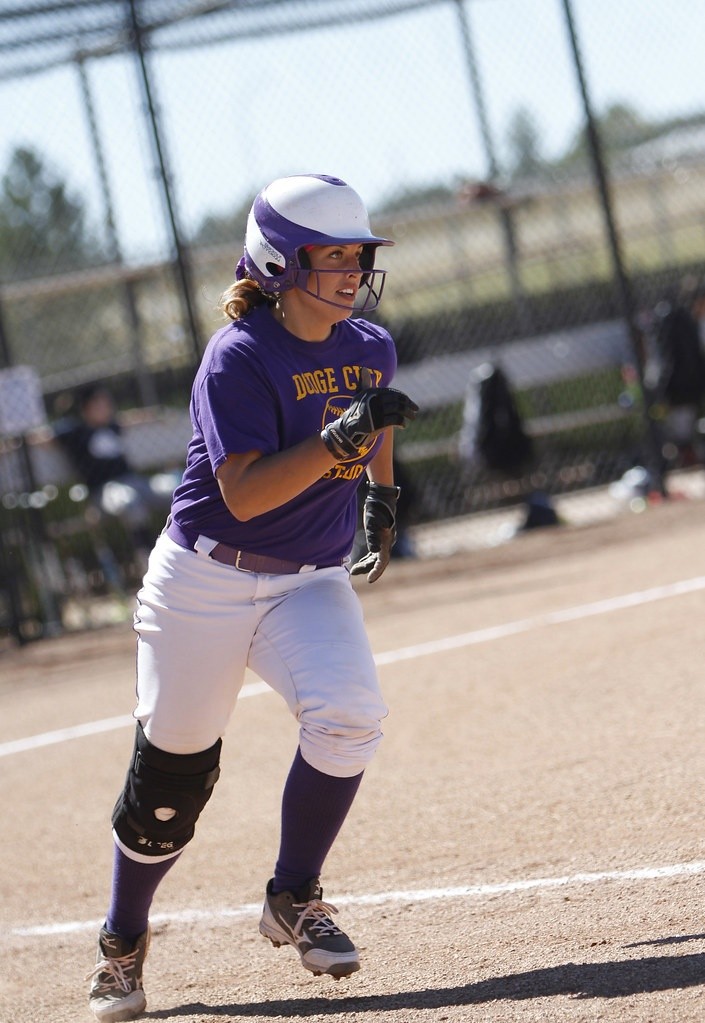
[350,482,402,583]
[319,387,421,463]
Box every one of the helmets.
[236,175,394,293]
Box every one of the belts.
[166,520,343,575]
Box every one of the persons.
[75,390,165,542]
[468,369,557,530]
[634,278,705,495]
[87,175,422,1023]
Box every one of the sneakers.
[84,924,149,1023]
[256,876,359,981]
